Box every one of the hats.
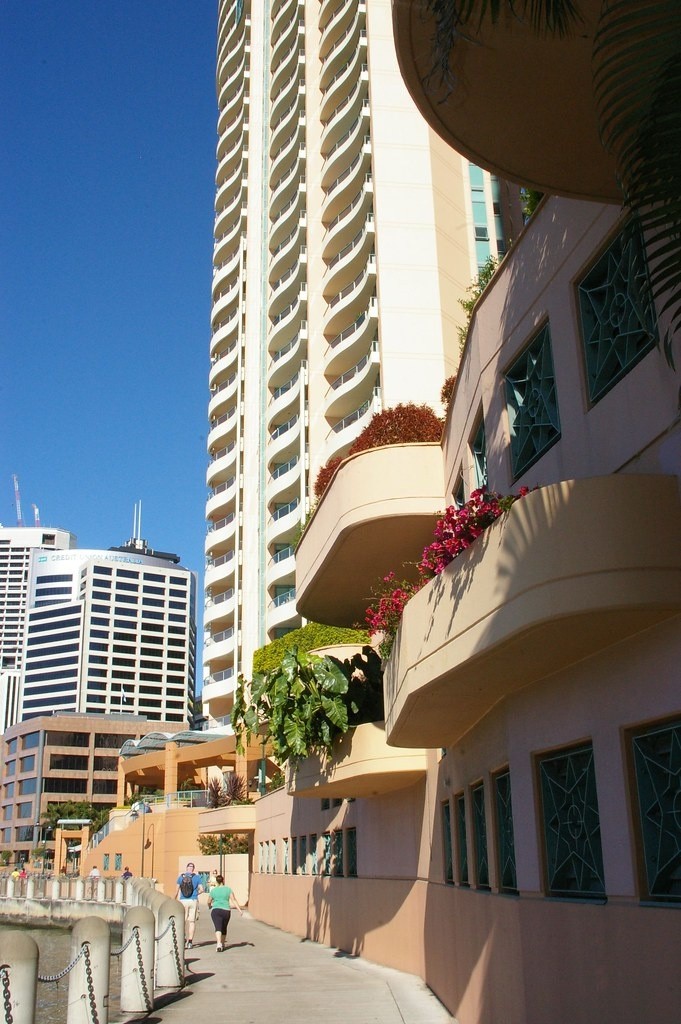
[187,863,195,867]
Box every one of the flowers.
[362,485,541,668]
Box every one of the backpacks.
[180,873,198,898]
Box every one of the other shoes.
[187,942,194,949]
[185,942,187,948]
[216,943,225,952]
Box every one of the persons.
[207,875,243,952]
[121,866,132,880]
[209,871,220,890]
[175,863,204,949]
[12,867,27,880]
[144,802,151,813]
[89,865,100,877]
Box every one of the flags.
[122,690,126,702]
